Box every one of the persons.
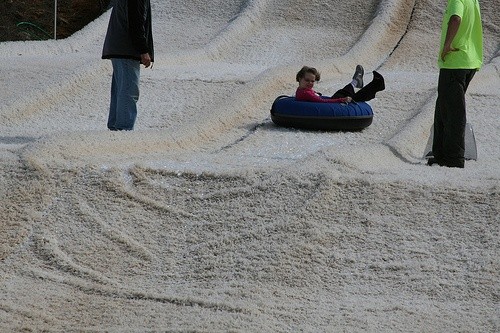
[296,64,386,104]
[101,0,154,131]
[425,0,484,169]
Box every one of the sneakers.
[373,70,385,91]
[352,64,365,88]
